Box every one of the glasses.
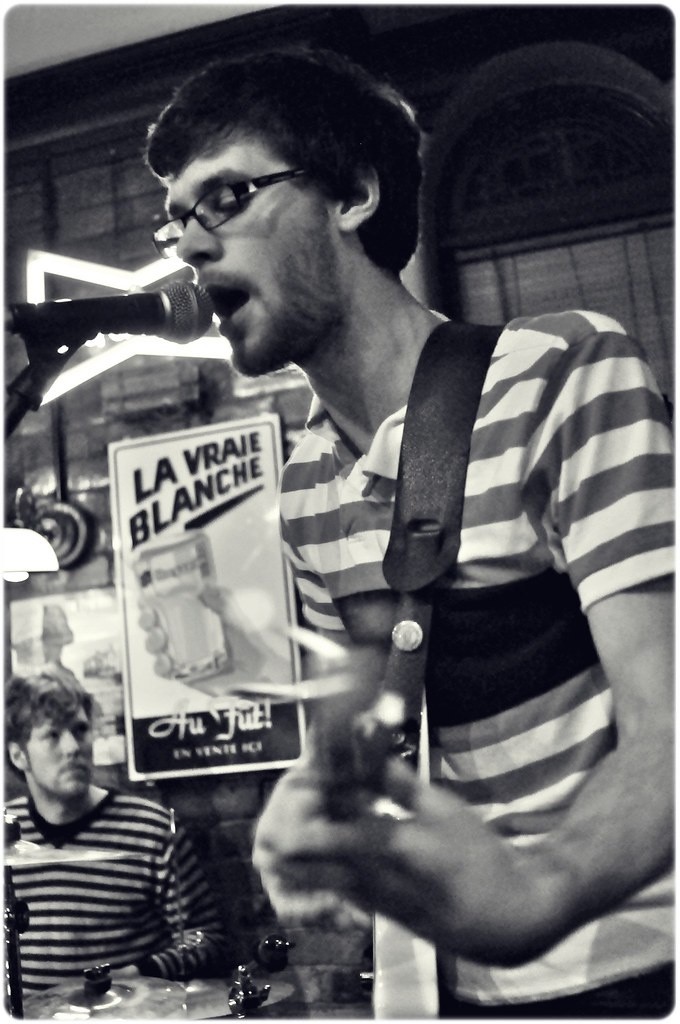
[148,164,306,259]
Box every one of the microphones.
[5,280,213,345]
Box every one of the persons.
[4,668,224,1013]
[138,587,292,704]
[146,50,676,1019]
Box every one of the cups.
[138,535,230,685]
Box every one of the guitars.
[316,645,444,1020]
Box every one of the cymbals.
[11,962,189,1021]
[177,975,297,1019]
